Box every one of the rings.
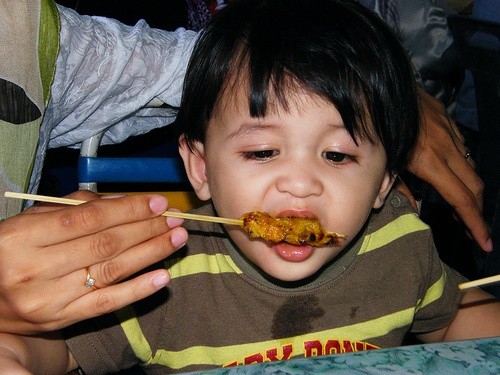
[465,151,471,159]
[85,267,99,291]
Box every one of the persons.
[1,0,500,375]
[0,0,493,335]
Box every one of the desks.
[168,331,500,375]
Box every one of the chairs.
[69,102,218,230]
